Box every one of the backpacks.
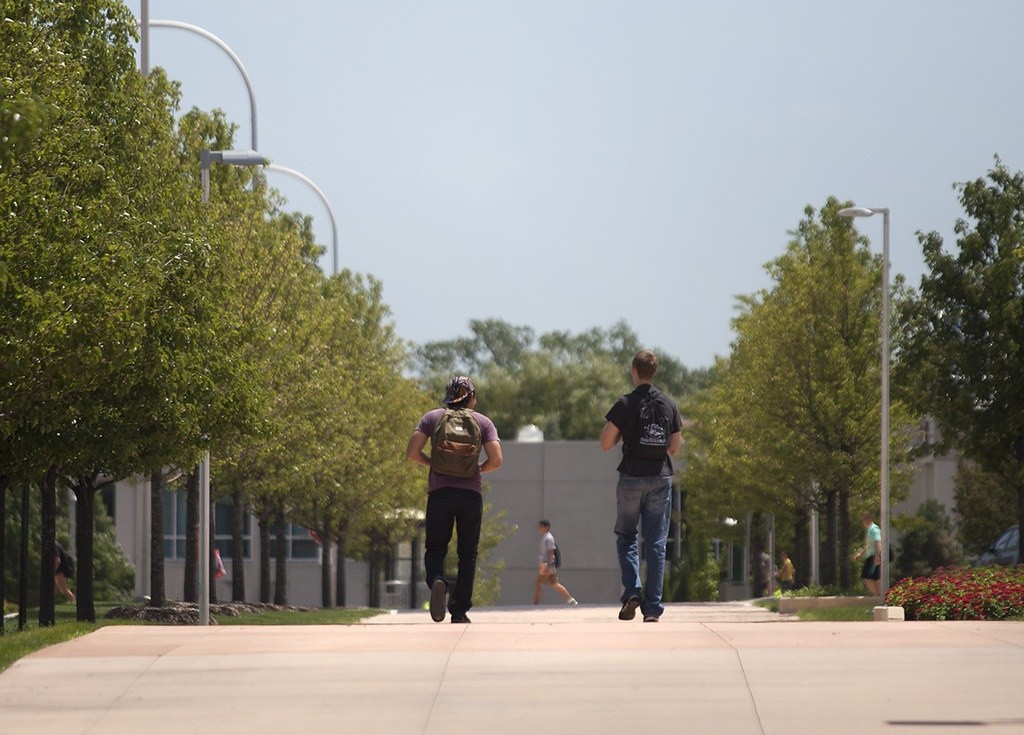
[631,388,671,461]
[432,405,481,478]
[554,542,561,568]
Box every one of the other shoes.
[430,579,446,621]
[568,597,578,605]
[619,595,641,620]
[452,610,472,623]
[643,616,659,622]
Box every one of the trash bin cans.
[381,580,407,609]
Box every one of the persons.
[852,511,882,595]
[534,520,578,606]
[778,550,795,594]
[407,376,503,623]
[749,542,778,599]
[601,351,681,622]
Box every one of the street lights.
[194,147,268,626]
[837,204,891,606]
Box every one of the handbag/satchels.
[869,555,881,579]
[889,546,895,563]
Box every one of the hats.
[441,376,475,403]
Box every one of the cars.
[974,521,1024,569]
